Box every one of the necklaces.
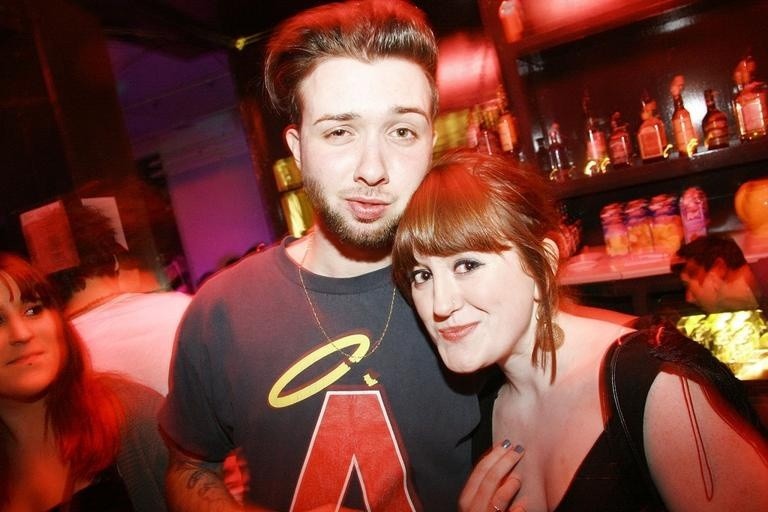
[294,227,400,362]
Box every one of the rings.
[488,497,504,512]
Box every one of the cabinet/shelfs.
[482,1,768,316]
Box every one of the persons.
[670,233,766,334]
[0,254,246,509]
[27,198,196,399]
[149,0,515,512]
[386,143,767,512]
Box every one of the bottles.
[474,55,768,185]
